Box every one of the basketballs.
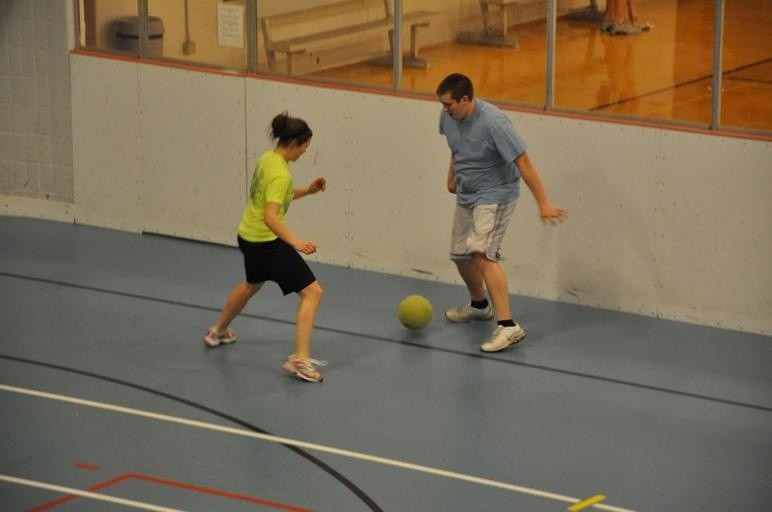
[399,293,433,329]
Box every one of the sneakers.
[282,354,323,383]
[444,300,496,323]
[203,326,238,348]
[480,322,526,352]
[600,16,643,35]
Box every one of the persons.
[202,113,326,384]
[600,0,653,36]
[435,71,567,354]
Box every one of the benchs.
[457,1,606,47]
[261,0,443,73]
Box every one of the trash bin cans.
[112,16,164,57]
